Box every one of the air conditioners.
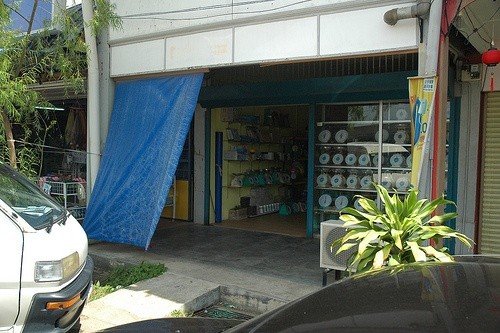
[319,219,375,271]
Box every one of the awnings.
[80,73,204,268]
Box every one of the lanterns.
[481,45,500,92]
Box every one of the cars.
[94,254,500,333]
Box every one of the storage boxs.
[221,108,240,121]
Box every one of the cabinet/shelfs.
[41,179,87,222]
[312,120,413,239]
[223,121,308,222]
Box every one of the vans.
[0,159,94,333]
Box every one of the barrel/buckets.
[278,199,306,216]
[248,206,257,216]
[240,197,250,207]
